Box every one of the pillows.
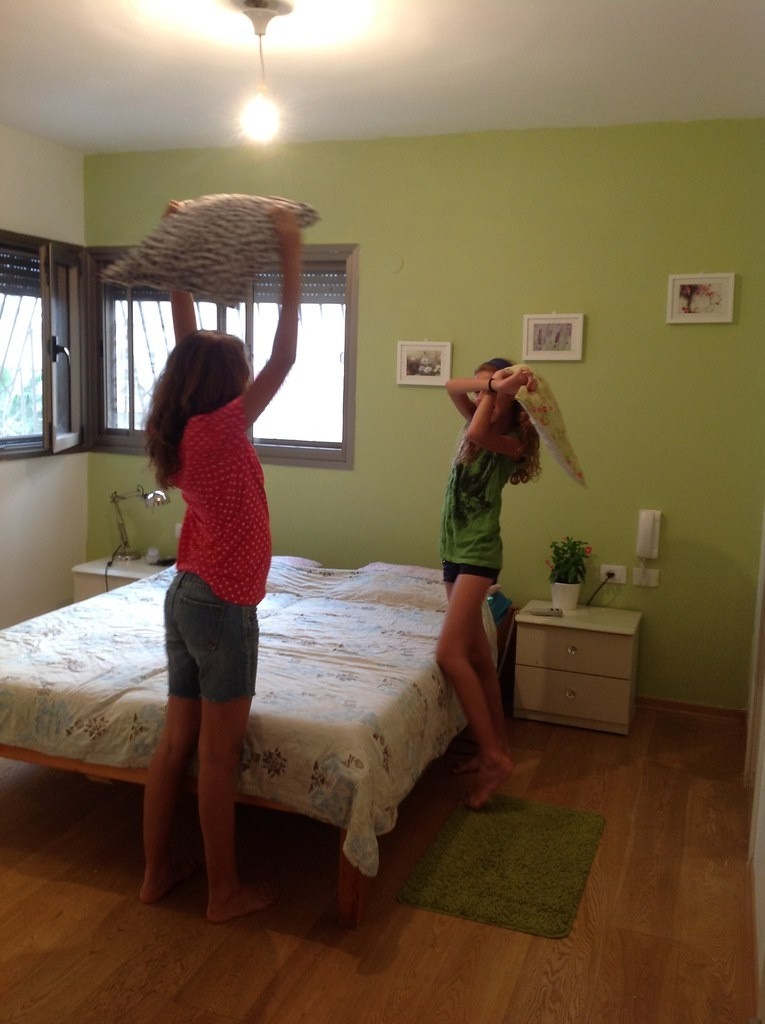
[358,559,503,597]
[98,193,322,310]
[270,555,323,567]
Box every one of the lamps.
[111,483,171,562]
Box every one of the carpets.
[396,793,606,939]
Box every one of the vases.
[551,582,582,611]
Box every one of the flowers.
[545,535,592,584]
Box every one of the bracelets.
[488,378,494,392]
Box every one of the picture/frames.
[666,272,735,324]
[523,313,585,361]
[396,340,452,386]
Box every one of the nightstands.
[512,600,642,737]
[71,557,170,602]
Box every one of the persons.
[434,356,540,808]
[138,199,302,924]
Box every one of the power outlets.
[599,564,627,585]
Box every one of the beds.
[0,556,511,931]
